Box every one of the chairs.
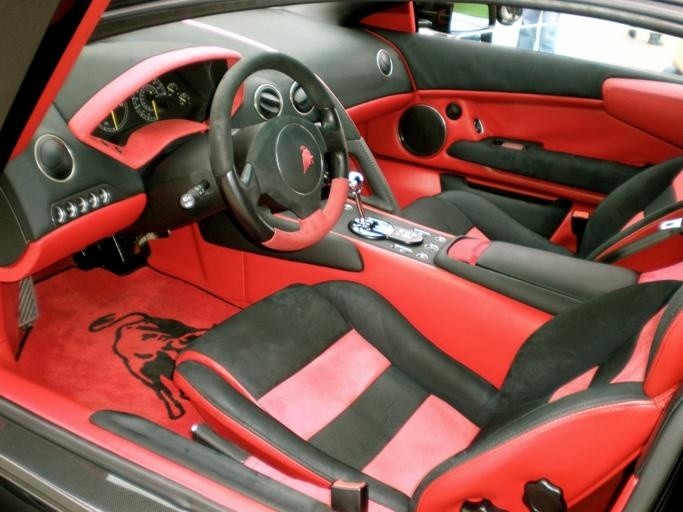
[400,156,683,313]
[172,279,682,512]
